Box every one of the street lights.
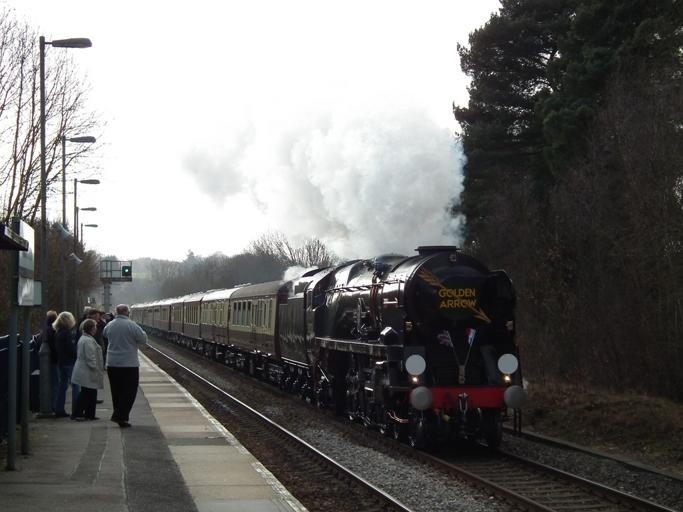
[60,131,100,315]
[35,33,95,419]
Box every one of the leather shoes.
[96,400,103,403]
[36,409,98,421]
[110,416,130,427]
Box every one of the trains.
[128,244,533,460]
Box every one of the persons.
[101,303,148,427]
[35,306,116,421]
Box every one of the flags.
[465,328,477,347]
[437,328,454,348]
